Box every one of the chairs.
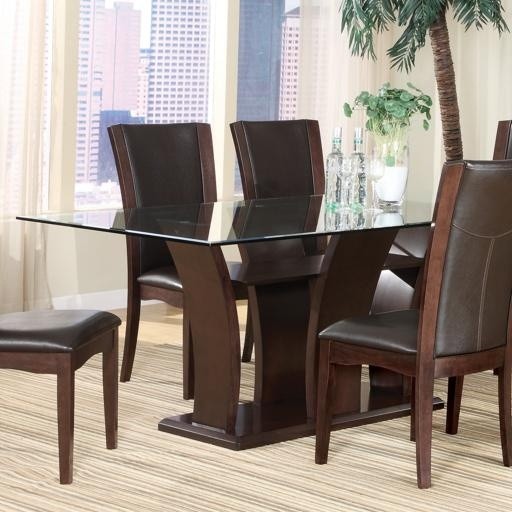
[228,117,327,364]
[1,307,124,486]
[107,122,250,400]
[314,159,512,488]
[443,120,511,437]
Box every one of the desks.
[15,194,447,451]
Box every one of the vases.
[371,201,405,230]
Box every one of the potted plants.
[343,81,432,206]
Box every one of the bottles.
[344,127,367,213]
[323,128,346,214]
